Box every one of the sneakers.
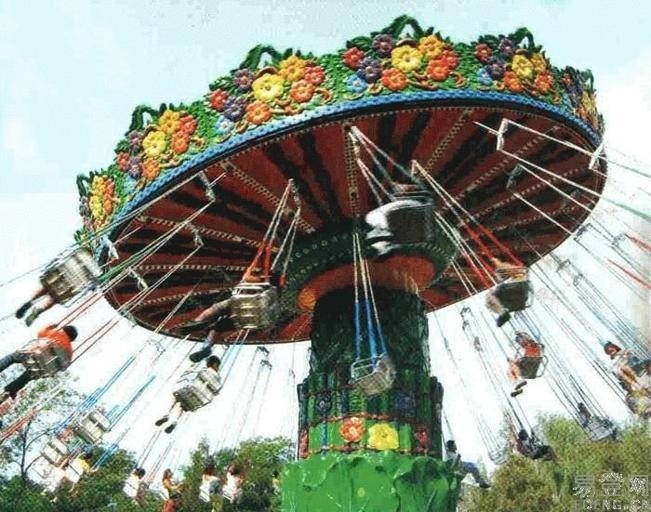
[155,415,177,434]
[178,320,208,333]
[191,346,213,363]
[16,301,40,327]
[496,313,513,327]
[510,381,527,396]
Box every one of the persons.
[65,450,284,510]
[505,330,541,397]
[179,268,286,362]
[363,176,428,261]
[0,324,79,403]
[15,248,98,326]
[444,343,650,488]
[485,254,527,326]
[155,354,224,434]
